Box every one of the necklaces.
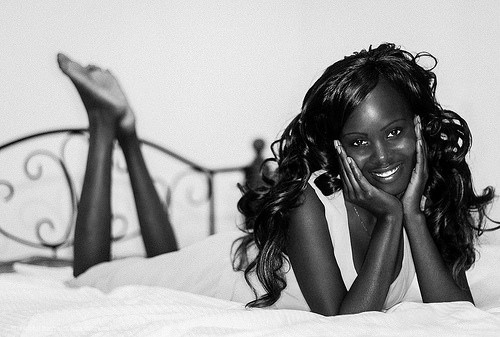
[350,203,373,237]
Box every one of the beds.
[1,127,498,337]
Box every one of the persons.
[56,40,500,316]
